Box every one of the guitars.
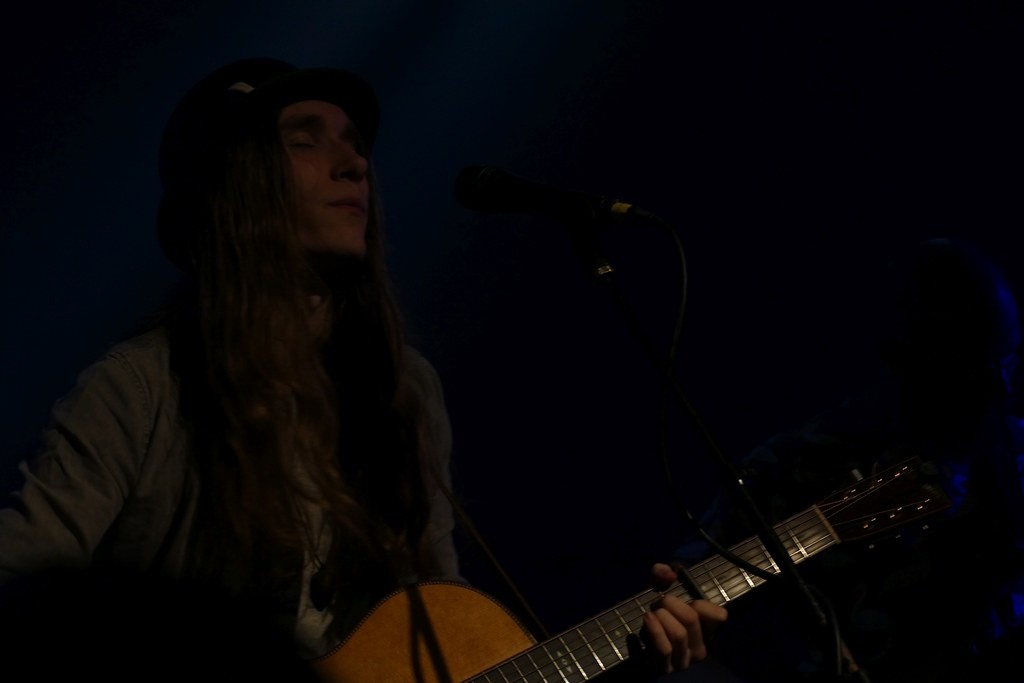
[304,447,967,683]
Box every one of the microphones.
[452,164,666,230]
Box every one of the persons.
[0,57,729,683]
[654,235,1024,681]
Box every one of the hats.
[154,58,379,164]
